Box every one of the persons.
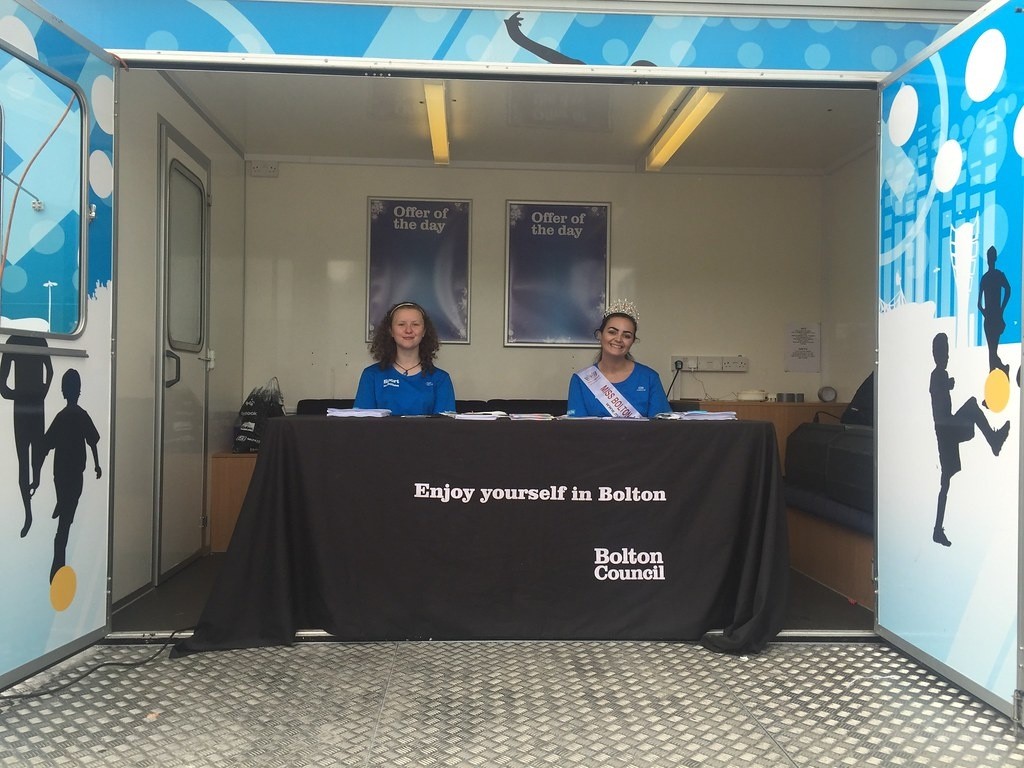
[353,301,457,416]
[567,312,673,419]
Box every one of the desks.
[169,414,791,660]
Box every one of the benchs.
[297,399,701,417]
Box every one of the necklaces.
[392,359,422,376]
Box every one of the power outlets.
[671,357,749,372]
[251,160,279,177]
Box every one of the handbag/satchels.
[232,377,287,454]
